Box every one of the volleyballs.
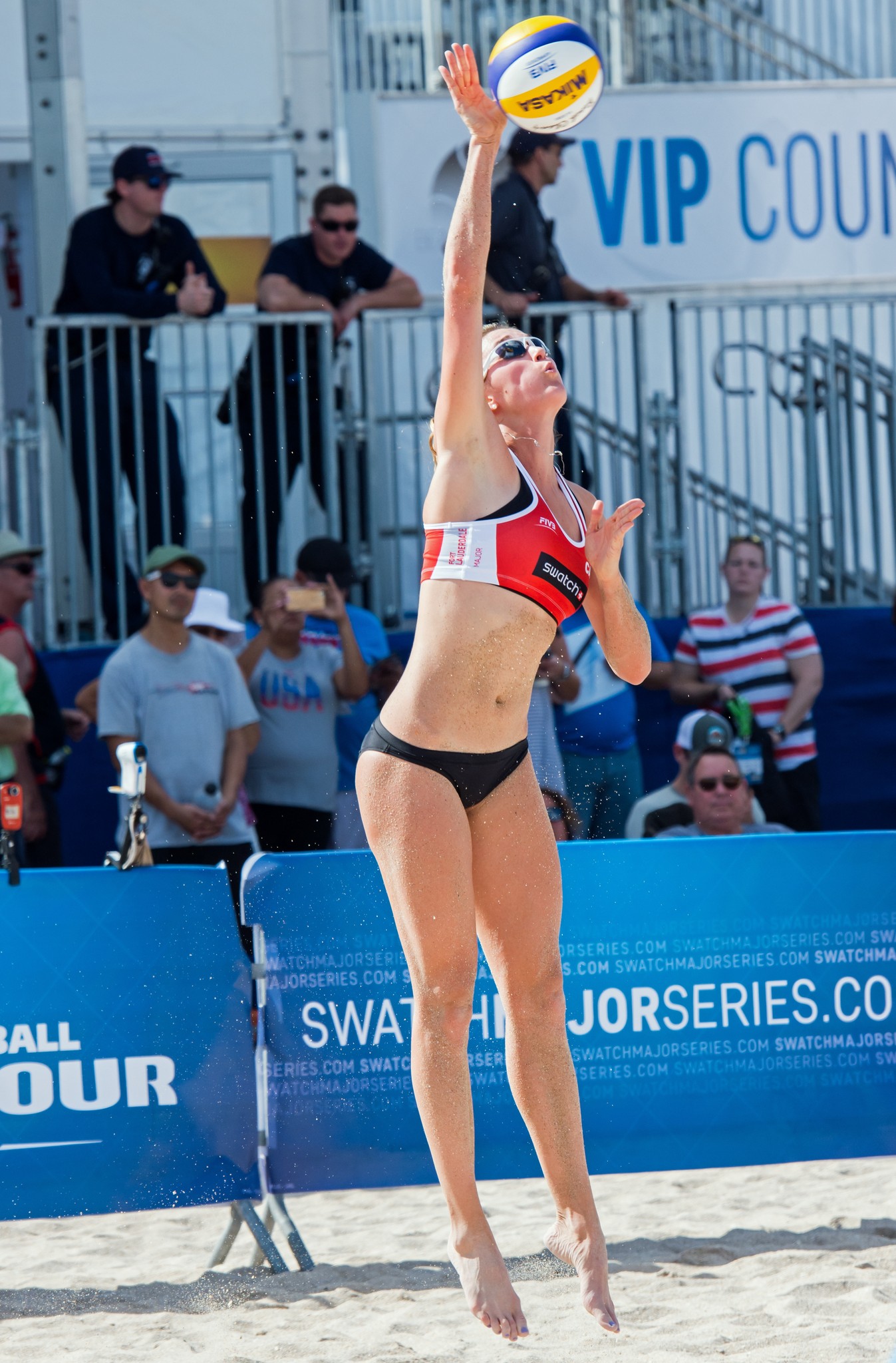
[487,15,605,134]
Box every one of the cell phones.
[285,584,326,613]
[0,783,23,830]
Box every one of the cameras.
[108,741,148,797]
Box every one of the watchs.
[775,722,789,742]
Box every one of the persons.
[624,708,766,838]
[216,183,424,632]
[668,532,824,832]
[232,573,369,852]
[480,128,633,504]
[525,597,673,841]
[354,40,654,1337]
[655,749,793,838]
[0,526,247,868]
[96,544,275,1053]
[47,146,226,643]
[226,535,404,852]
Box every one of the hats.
[0,530,45,563]
[296,536,359,588]
[184,588,244,633]
[676,707,733,753]
[509,128,575,157]
[141,543,209,576]
[113,147,183,182]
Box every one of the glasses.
[318,219,359,233]
[6,561,36,577]
[483,336,551,383]
[144,176,169,190]
[146,568,201,590]
[699,773,743,791]
